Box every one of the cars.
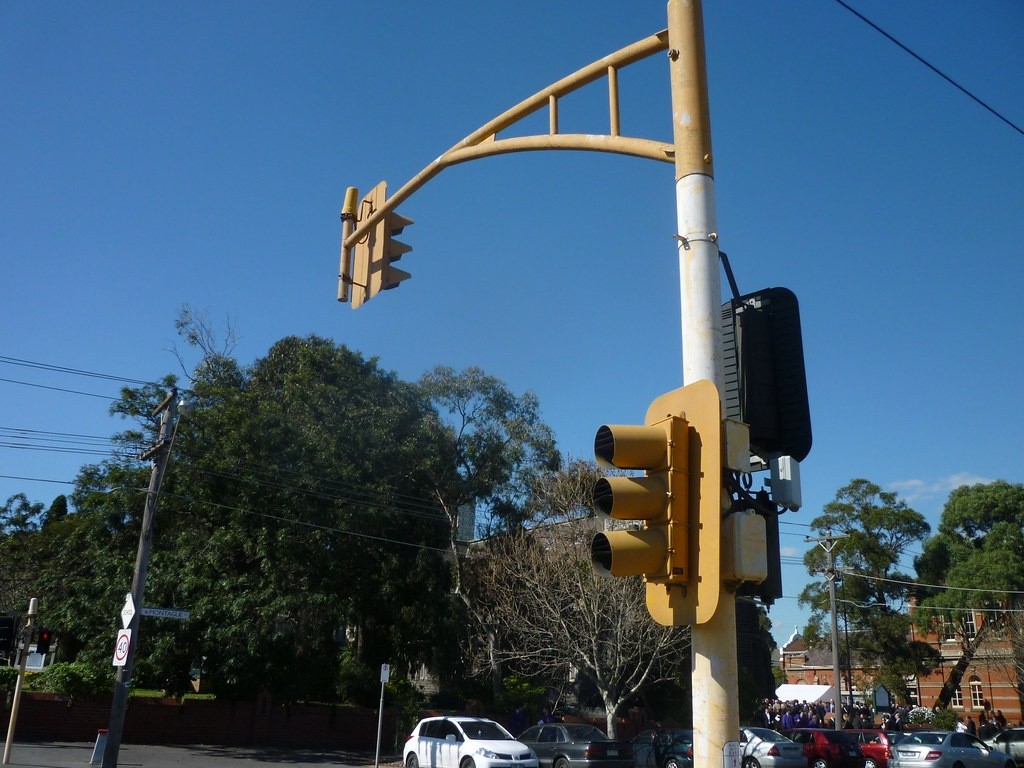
[630,727,693,768]
[518,723,635,768]
[887,731,1015,768]
[401,716,540,768]
[782,727,853,768]
[687,726,808,768]
[974,727,1023,763]
[842,729,892,768]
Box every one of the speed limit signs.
[113,628,132,666]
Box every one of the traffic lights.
[36,627,52,655]
[593,377,723,629]
[350,180,414,311]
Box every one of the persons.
[755,697,1023,753]
[538,709,564,740]
